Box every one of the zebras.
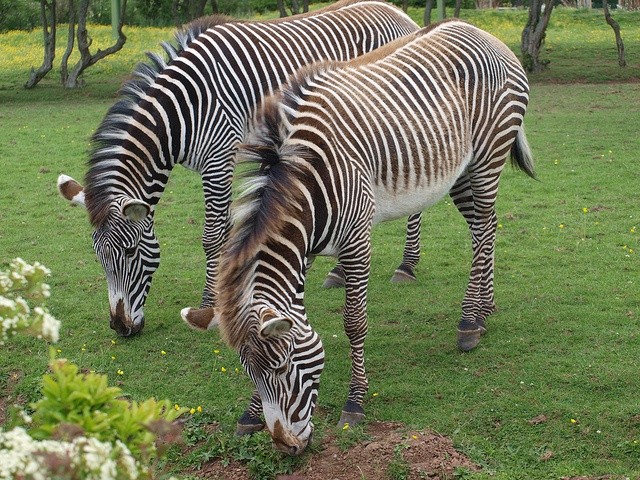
[56,0,423,338]
[180,16,543,457]
